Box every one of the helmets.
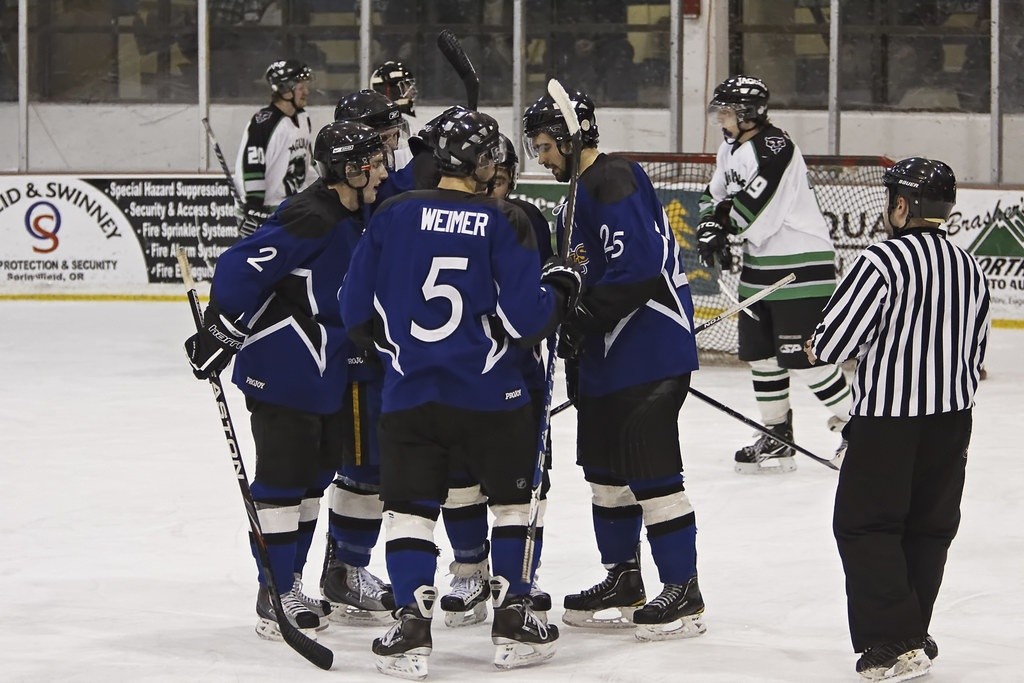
[433,109,519,199]
[315,59,417,187]
[707,76,769,128]
[266,60,316,94]
[880,155,956,219]
[522,89,599,160]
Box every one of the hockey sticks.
[175,245,335,672]
[520,77,583,585]
[203,113,256,231]
[686,385,849,472]
[434,29,482,112]
[549,273,798,417]
[710,255,761,322]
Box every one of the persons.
[838,0,1024,114]
[205,0,264,96]
[521,88,708,642]
[368,0,671,108]
[132,0,159,102]
[184,59,561,679]
[802,157,992,683]
[696,75,857,474]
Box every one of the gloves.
[238,202,274,240]
[556,302,604,366]
[185,303,251,379]
[694,214,733,271]
[407,105,465,158]
[541,254,583,308]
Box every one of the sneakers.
[319,533,401,626]
[489,575,558,669]
[255,575,320,645]
[632,551,707,642]
[828,416,849,467]
[372,584,438,681]
[291,572,334,630]
[525,561,551,626]
[562,547,646,628]
[735,409,797,475]
[439,539,492,628]
[854,634,938,683]
